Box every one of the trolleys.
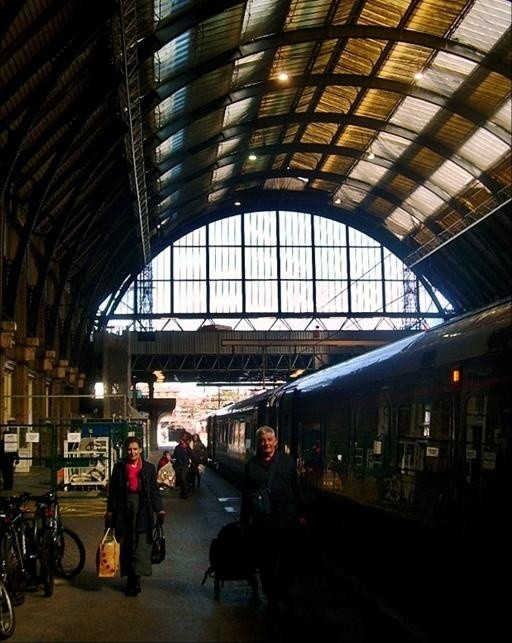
[198,519,263,598]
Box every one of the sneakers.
[126,577,141,596]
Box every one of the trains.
[206,295,512,586]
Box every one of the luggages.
[201,521,268,594]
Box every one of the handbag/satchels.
[245,489,272,517]
[151,538,165,563]
[96,541,121,579]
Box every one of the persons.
[105,435,168,598]
[190,433,208,488]
[171,431,197,499]
[156,449,171,491]
[239,424,308,617]
[1,429,21,491]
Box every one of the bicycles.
[0,479,85,642]
